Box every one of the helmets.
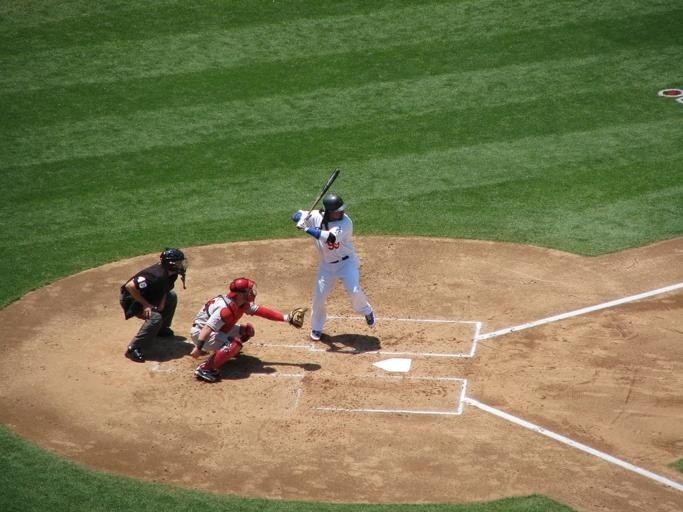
[228,279,257,302]
[323,193,346,213]
[160,248,187,274]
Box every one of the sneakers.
[129,346,144,362]
[311,329,321,340]
[158,328,173,337]
[365,312,375,326]
[195,367,220,382]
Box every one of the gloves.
[296,211,312,232]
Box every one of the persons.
[189,277,304,383]
[291,193,376,341]
[119,247,184,362]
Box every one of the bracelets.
[196,340,205,350]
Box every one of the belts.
[331,256,348,264]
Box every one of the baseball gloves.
[288,308,309,328]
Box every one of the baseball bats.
[297,168,340,230]
